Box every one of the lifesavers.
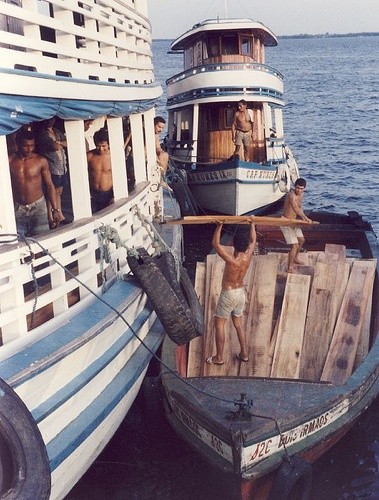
[152,252,204,337]
[276,164,291,193]
[127,247,196,346]
[287,158,301,184]
[0,377,52,499]
[169,175,200,217]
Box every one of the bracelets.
[51,208,60,212]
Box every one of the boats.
[166,16,303,223]
[159,210,379,488]
[0,0,169,500]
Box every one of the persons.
[39,116,73,230]
[86,127,114,215]
[7,130,60,240]
[280,178,312,274]
[232,100,255,162]
[124,116,166,166]
[205,215,257,365]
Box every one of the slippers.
[206,356,223,365]
[236,353,249,362]
